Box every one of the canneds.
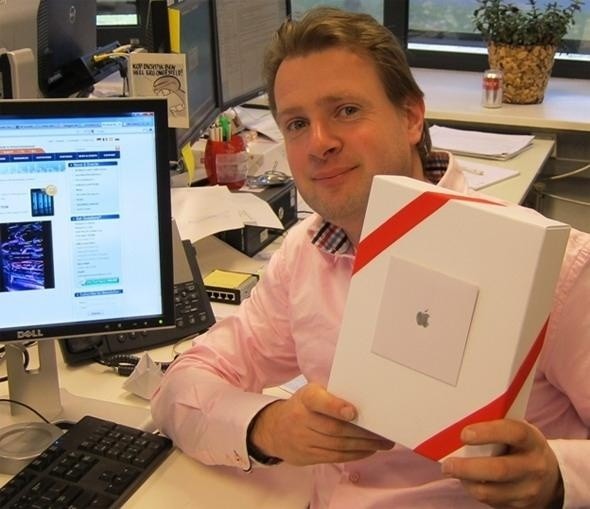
[482,69,504,108]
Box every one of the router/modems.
[202,268,260,304]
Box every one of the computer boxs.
[189,174,297,257]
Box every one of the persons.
[149,7,590,509]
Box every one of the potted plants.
[470,0,586,104]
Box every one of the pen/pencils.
[209,116,231,143]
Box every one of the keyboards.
[0,415,172,509]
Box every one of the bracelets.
[247,411,284,466]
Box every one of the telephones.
[59,218,216,368]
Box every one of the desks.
[0,104,562,509]
[246,61,590,134]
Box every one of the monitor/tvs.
[168,0,222,188]
[211,0,292,143]
[0,96,176,436]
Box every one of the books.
[428,121,536,161]
[456,159,521,192]
